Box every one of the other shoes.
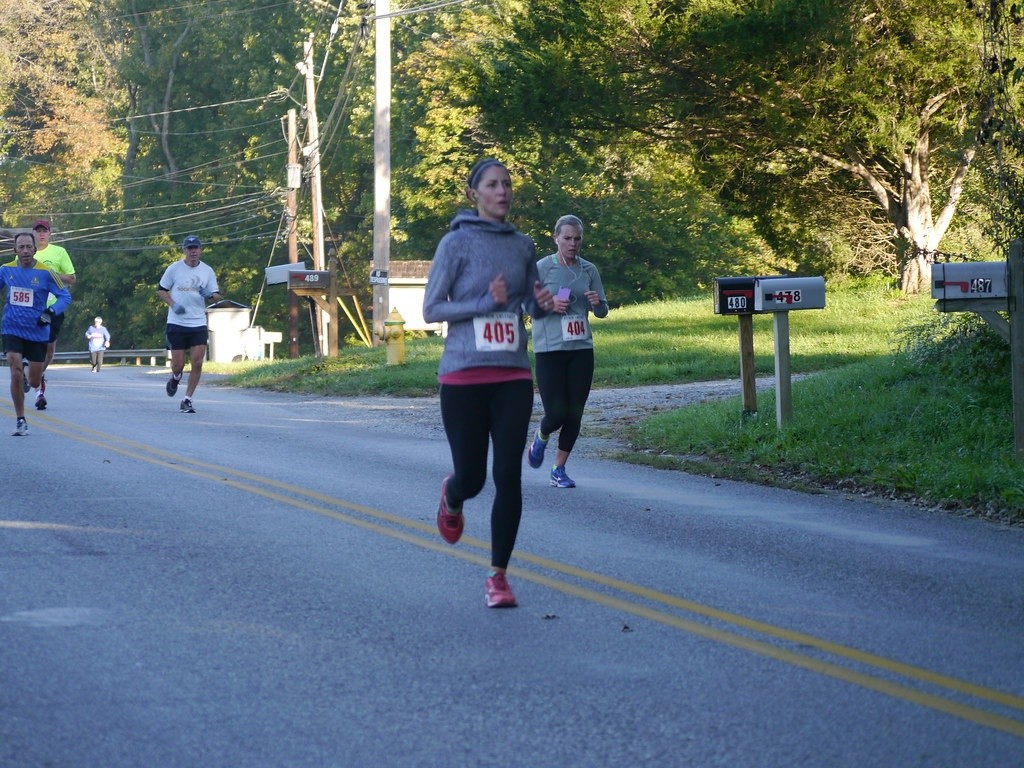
[92,367,97,373]
[41,375,48,391]
[35,394,47,411]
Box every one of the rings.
[544,301,549,306]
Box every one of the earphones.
[556,239,558,244]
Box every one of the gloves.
[199,288,213,299]
[37,309,53,328]
[171,302,186,315]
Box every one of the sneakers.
[179,398,196,414]
[166,373,182,397]
[10,418,30,436]
[484,568,516,608]
[437,475,465,544]
[550,466,576,488]
[527,428,549,468]
[22,360,31,393]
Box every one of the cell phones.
[557,286,571,300]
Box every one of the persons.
[85,316,111,373]
[526,215,608,487]
[157,236,220,413]
[0,233,71,437]
[14,220,77,409]
[423,158,556,608]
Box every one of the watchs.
[48,307,55,315]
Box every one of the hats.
[33,220,50,230]
[184,235,201,248]
